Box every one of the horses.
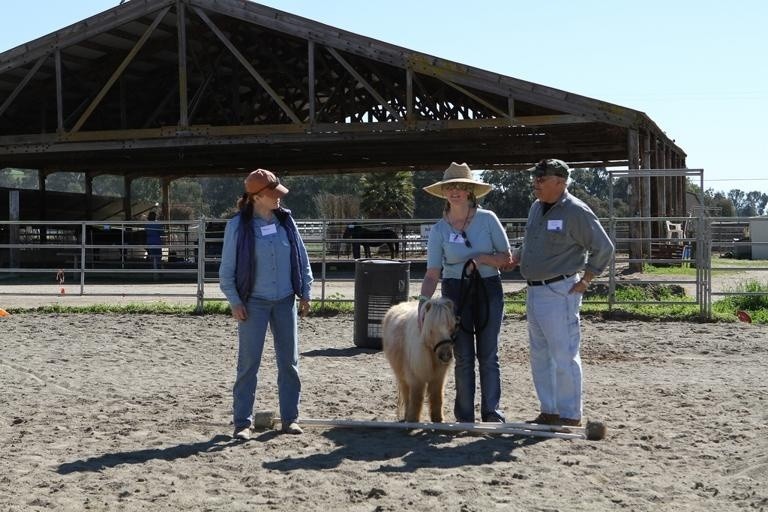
[343,226,400,261]
[81,226,127,260]
[382,296,460,435]
[123,229,151,261]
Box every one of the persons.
[415,159,514,425]
[143,211,166,280]
[503,156,616,433]
[217,167,315,441]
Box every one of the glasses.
[460,227,473,249]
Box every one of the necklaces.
[445,209,470,241]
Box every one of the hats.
[422,160,496,200]
[243,168,291,199]
[524,158,571,178]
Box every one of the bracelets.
[580,277,590,289]
[418,293,432,305]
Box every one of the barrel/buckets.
[353,257,411,352]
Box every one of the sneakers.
[281,420,303,434]
[233,423,252,442]
[524,409,583,427]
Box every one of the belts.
[524,272,580,286]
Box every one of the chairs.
[666,221,683,248]
